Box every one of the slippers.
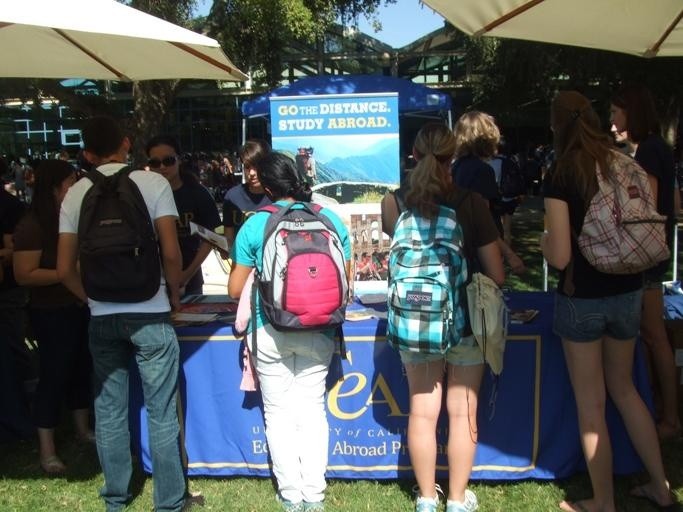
[39,455,69,476]
[558,496,616,512]
[76,428,97,442]
[624,483,674,506]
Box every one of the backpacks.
[574,143,673,277]
[385,190,470,353]
[256,198,352,334]
[75,163,161,307]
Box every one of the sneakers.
[413,484,440,512]
[275,493,327,512]
[653,419,682,439]
[445,489,479,511]
[183,494,205,510]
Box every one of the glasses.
[148,157,178,169]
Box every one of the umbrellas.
[420,0,683,59]
[0,0,250,83]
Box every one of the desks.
[130,292,682,482]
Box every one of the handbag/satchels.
[463,272,509,378]
